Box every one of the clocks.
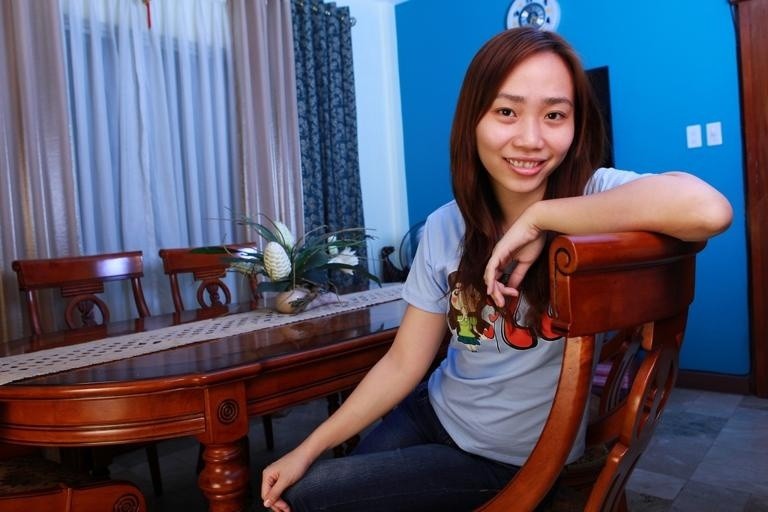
[500,1,563,36]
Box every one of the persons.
[259,27,734,512]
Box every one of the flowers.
[191,206,383,297]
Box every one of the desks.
[2,280,450,510]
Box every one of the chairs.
[157,244,276,450]
[480,222,711,510]
[11,251,164,492]
